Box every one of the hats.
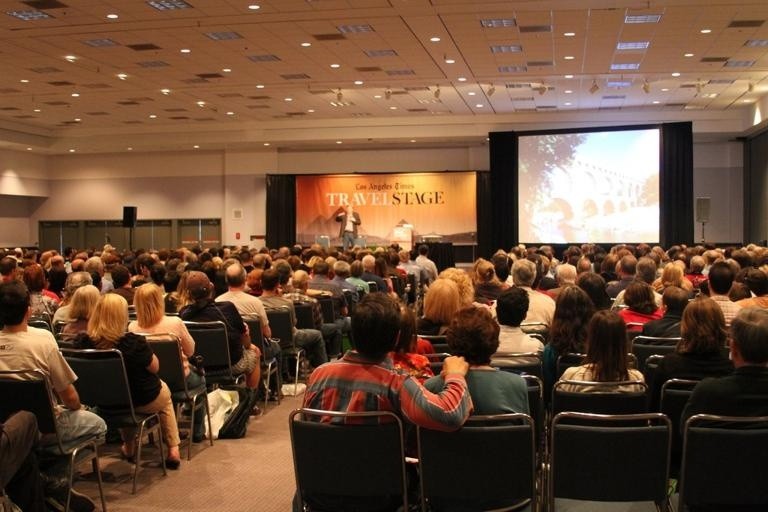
[186,271,211,291]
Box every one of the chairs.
[553,353,637,376]
[24,295,185,342]
[677,414,768,512]
[342,268,428,328]
[417,413,537,512]
[238,313,280,409]
[294,302,315,371]
[662,378,702,465]
[512,375,543,504]
[402,297,737,353]
[0,368,107,511]
[547,380,649,459]
[317,298,340,362]
[127,333,213,461]
[182,321,247,397]
[58,348,167,495]
[289,409,417,512]
[645,354,695,380]
[468,352,544,387]
[265,310,306,398]
[407,353,452,380]
[547,413,670,512]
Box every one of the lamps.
[696,196,715,249]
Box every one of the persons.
[546,264,576,299]
[699,259,752,302]
[52,272,92,335]
[579,274,613,312]
[0,410,47,512]
[599,254,618,282]
[127,282,206,442]
[48,256,68,297]
[647,298,729,424]
[62,285,100,340]
[73,294,181,470]
[408,251,429,288]
[730,250,752,280]
[492,254,515,290]
[0,281,108,511]
[557,311,646,394]
[178,270,262,422]
[414,244,439,283]
[679,310,768,433]
[24,265,60,324]
[684,256,707,282]
[735,270,767,310]
[618,282,663,332]
[651,246,669,268]
[636,244,651,260]
[84,257,113,294]
[540,246,559,274]
[119,246,271,274]
[492,259,557,334]
[165,292,183,318]
[676,261,742,356]
[281,270,342,362]
[605,256,637,298]
[247,269,263,296]
[652,260,693,298]
[536,250,554,279]
[416,267,473,337]
[388,301,440,386]
[309,261,355,348]
[495,287,545,357]
[524,254,557,301]
[213,264,284,402]
[702,250,719,275]
[106,266,136,305]
[257,269,327,367]
[581,243,597,273]
[666,245,681,263]
[576,257,592,284]
[301,292,474,431]
[643,286,688,337]
[71,259,85,271]
[567,246,582,267]
[334,203,361,252]
[0,246,119,268]
[540,255,556,290]
[0,257,18,281]
[655,263,689,298]
[388,252,408,287]
[362,255,388,295]
[375,257,397,297]
[753,248,768,273]
[271,243,402,268]
[611,258,663,311]
[422,307,529,427]
[471,259,502,301]
[345,259,369,301]
[543,285,594,402]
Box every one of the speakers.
[697,197,710,224]
[123,207,138,228]
